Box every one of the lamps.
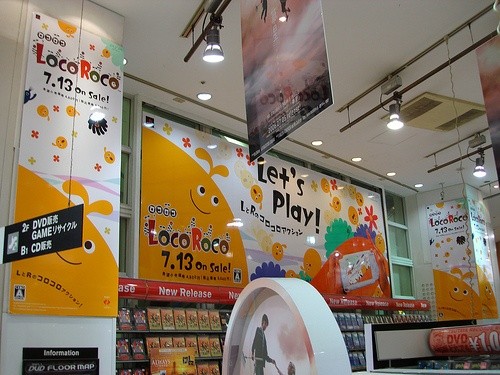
[387,99,404,130]
[203,25,224,62]
[472,157,486,178]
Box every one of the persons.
[276,362,296,375]
[251,313,276,375]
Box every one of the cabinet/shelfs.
[116,307,430,375]
